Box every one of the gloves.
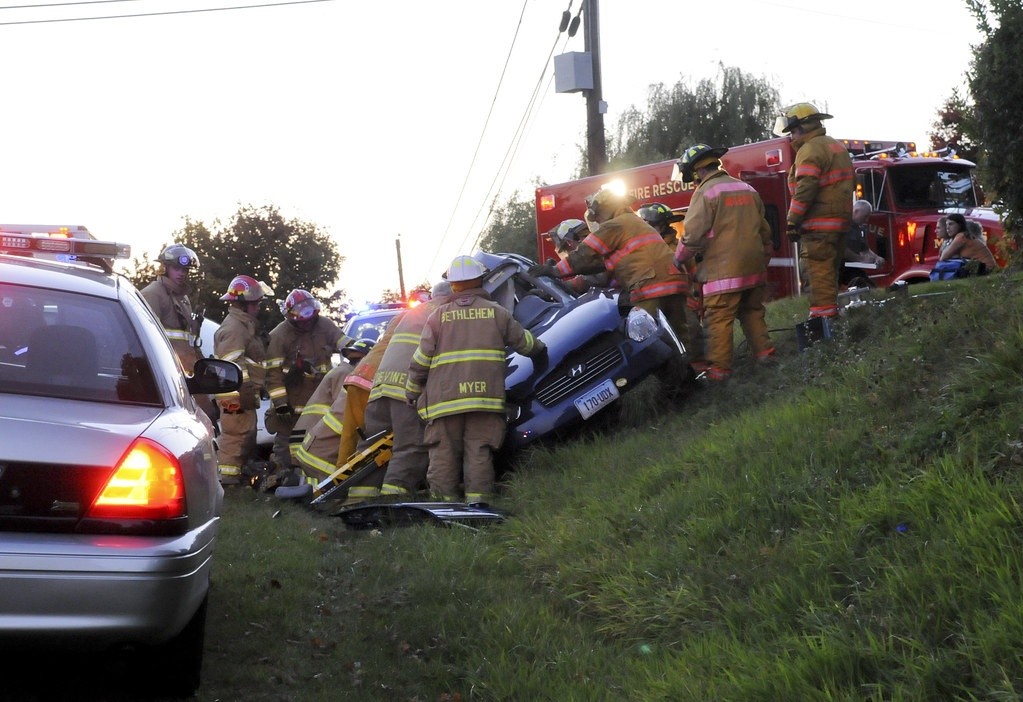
[275,403,293,425]
[534,339,545,354]
[673,256,690,272]
[528,256,562,278]
[786,221,802,241]
[239,381,258,411]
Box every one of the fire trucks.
[534,136,1017,305]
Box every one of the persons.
[139,244,221,439]
[673,144,775,380]
[839,200,884,287]
[935,213,998,276]
[781,103,857,322]
[214,255,548,517]
[527,189,704,400]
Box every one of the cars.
[216,352,351,460]
[0,233,244,702]
[469,251,701,476]
[1,223,114,271]
[340,303,407,366]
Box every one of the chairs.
[512,295,544,324]
[361,328,381,342]
[27,325,100,391]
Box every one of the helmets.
[554,219,587,253]
[587,188,637,223]
[676,143,728,183]
[431,282,453,298]
[637,201,686,228]
[152,244,200,269]
[281,289,323,332]
[219,275,276,312]
[782,103,833,133]
[341,338,376,359]
[442,255,490,282]
